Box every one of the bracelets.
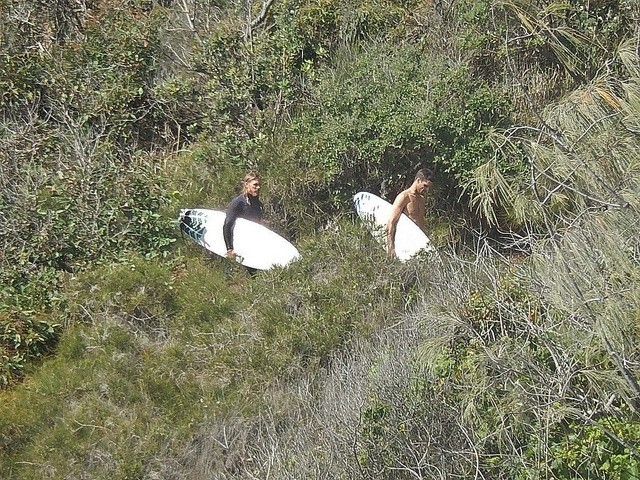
[227,249,233,253]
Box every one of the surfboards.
[353,192,443,266]
[176,208,303,271]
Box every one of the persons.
[386,168,435,265]
[223,171,265,282]
